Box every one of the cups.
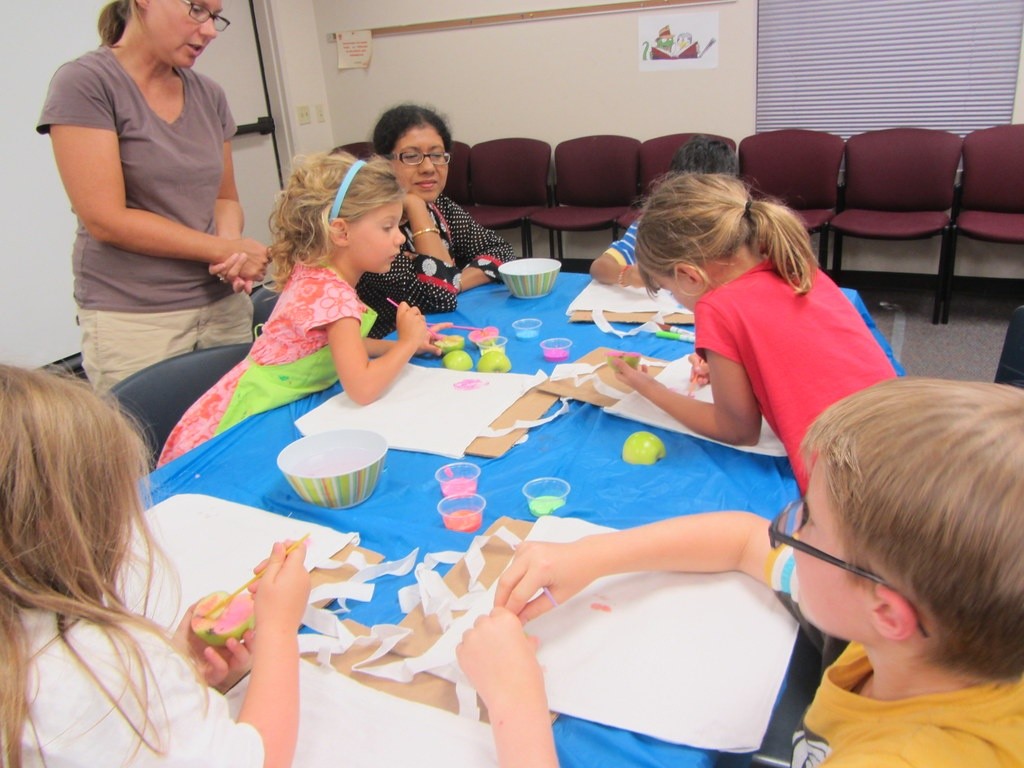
[512,318,542,341]
[477,336,508,355]
[522,477,570,517]
[437,494,486,532]
[435,464,481,498]
[540,338,572,361]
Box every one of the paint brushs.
[512,542,560,610]
[426,323,485,331]
[386,297,447,344]
[688,358,705,396]
[200,532,310,619]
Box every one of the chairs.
[249,282,281,342]
[737,128,845,275]
[101,342,255,474]
[613,134,737,242]
[939,123,1024,326]
[829,127,962,327]
[525,135,642,259]
[463,137,555,258]
[442,140,469,211]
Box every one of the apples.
[434,327,511,373]
[605,351,641,371]
[622,431,666,465]
[190,590,256,647]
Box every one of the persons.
[611,172,898,499]
[455,377,1024,768]
[0,363,312,768]
[36,0,275,394]
[155,153,453,474]
[589,133,739,289]
[355,102,515,338]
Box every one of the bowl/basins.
[277,430,388,509]
[499,257,561,299]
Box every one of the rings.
[219,275,224,281]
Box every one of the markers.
[656,331,696,344]
[654,321,695,336]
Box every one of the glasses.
[381,149,451,166]
[182,0,230,32]
[767,497,928,640]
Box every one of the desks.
[109,272,905,768]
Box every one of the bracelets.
[412,228,440,239]
[618,265,629,287]
[459,279,463,292]
